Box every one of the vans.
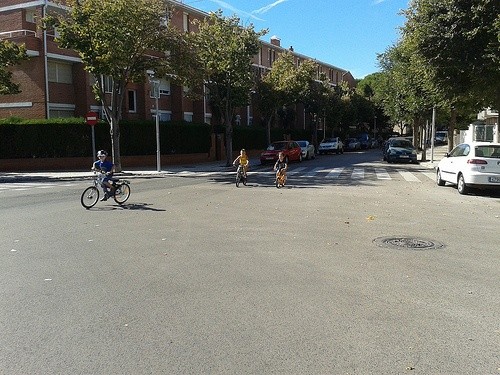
[436,130,448,145]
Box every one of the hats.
[98,150,107,157]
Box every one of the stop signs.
[86,111,98,125]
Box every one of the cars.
[342,135,413,152]
[296,140,316,161]
[386,138,417,164]
[319,137,344,155]
[260,141,303,167]
[436,141,500,197]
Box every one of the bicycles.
[234,164,247,187]
[80,168,130,208]
[274,168,286,188]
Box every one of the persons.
[233,149,249,178]
[273,152,287,183]
[92,150,115,201]
[378,134,384,149]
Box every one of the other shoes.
[110,187,116,194]
[101,196,107,201]
